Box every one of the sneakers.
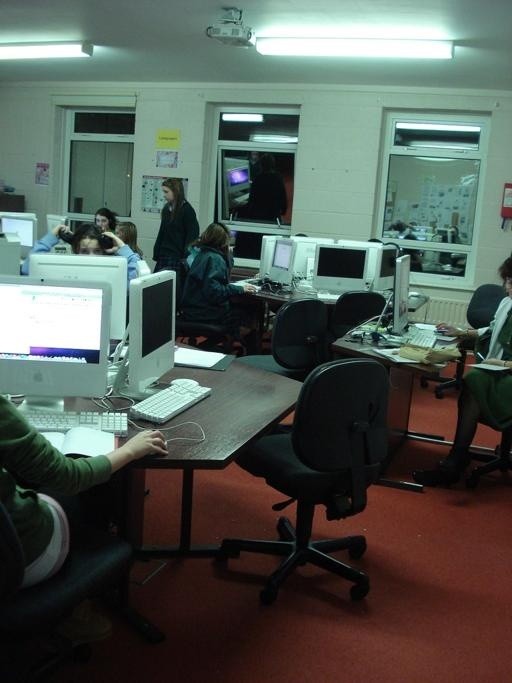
[38,613,113,651]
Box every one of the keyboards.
[316,294,340,300]
[18,411,129,438]
[409,331,437,348]
[130,383,212,423]
[234,193,250,202]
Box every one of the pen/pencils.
[477,351,486,361]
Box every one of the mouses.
[434,328,448,333]
[170,378,199,386]
[145,436,168,456]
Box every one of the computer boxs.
[61,460,145,560]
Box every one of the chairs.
[0,467,166,683]
[158,266,232,353]
[235,299,329,381]
[470,427,512,478]
[420,284,508,399]
[215,357,388,606]
[331,291,387,338]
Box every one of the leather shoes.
[412,470,461,487]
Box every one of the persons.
[399,235,423,271]
[411,256,511,487]
[21,207,143,297]
[151,178,199,299]
[179,221,261,356]
[0,391,170,648]
[240,153,288,225]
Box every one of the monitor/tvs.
[113,270,177,400]
[0,211,129,340]
[0,273,112,411]
[382,225,451,255]
[224,158,250,193]
[259,234,399,293]
[375,254,410,343]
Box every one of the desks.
[230,277,429,341]
[0,341,306,561]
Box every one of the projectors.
[205,24,256,46]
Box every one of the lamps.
[255,37,454,59]
[0,40,93,60]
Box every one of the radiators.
[408,297,473,330]
